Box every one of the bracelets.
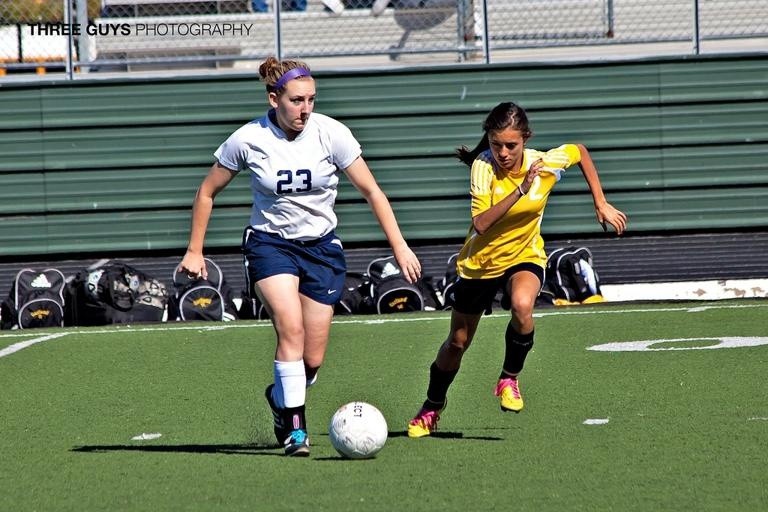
[518,185,526,196]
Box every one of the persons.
[406,100,627,438]
[178,58,422,456]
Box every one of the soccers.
[328,402,388,460]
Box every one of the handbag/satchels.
[337,256,456,315]
[542,245,602,303]
[0,257,268,330]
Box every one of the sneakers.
[266,383,285,445]
[496,377,523,413]
[408,398,447,439]
[283,430,311,457]
